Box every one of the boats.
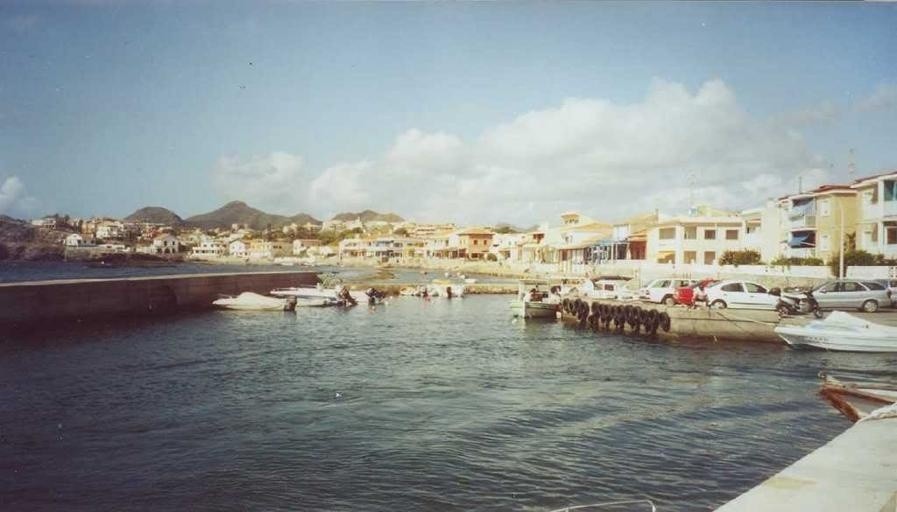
[399,284,462,298]
[270,285,386,308]
[773,310,896,353]
[213,291,297,312]
[510,277,633,319]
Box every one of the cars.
[811,278,896,313]
[639,279,782,310]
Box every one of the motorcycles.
[769,287,824,319]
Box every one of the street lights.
[800,191,843,278]
